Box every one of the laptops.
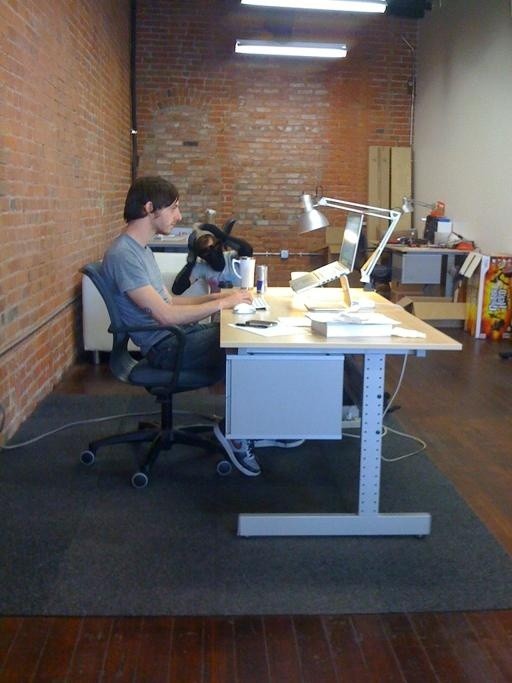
[289,211,365,293]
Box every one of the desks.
[215,288,462,534]
[370,239,478,296]
[143,227,193,252]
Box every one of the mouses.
[234,304,255,313]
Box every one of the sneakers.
[254,440,304,449]
[213,417,262,477]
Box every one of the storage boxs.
[396,295,466,328]
[309,226,343,262]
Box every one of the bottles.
[256,266,267,294]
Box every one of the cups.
[232,256,256,291]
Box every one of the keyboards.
[249,292,270,312]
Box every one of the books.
[309,318,392,339]
[304,310,402,327]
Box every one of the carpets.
[0,391,512,615]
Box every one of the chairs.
[77,260,232,490]
[354,225,391,291]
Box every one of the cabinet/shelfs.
[363,146,413,249]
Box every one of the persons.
[101,173,306,478]
[171,218,253,322]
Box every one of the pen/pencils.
[236,324,267,328]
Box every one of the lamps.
[401,196,445,218]
[295,193,403,291]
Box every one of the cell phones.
[246,320,277,329]
